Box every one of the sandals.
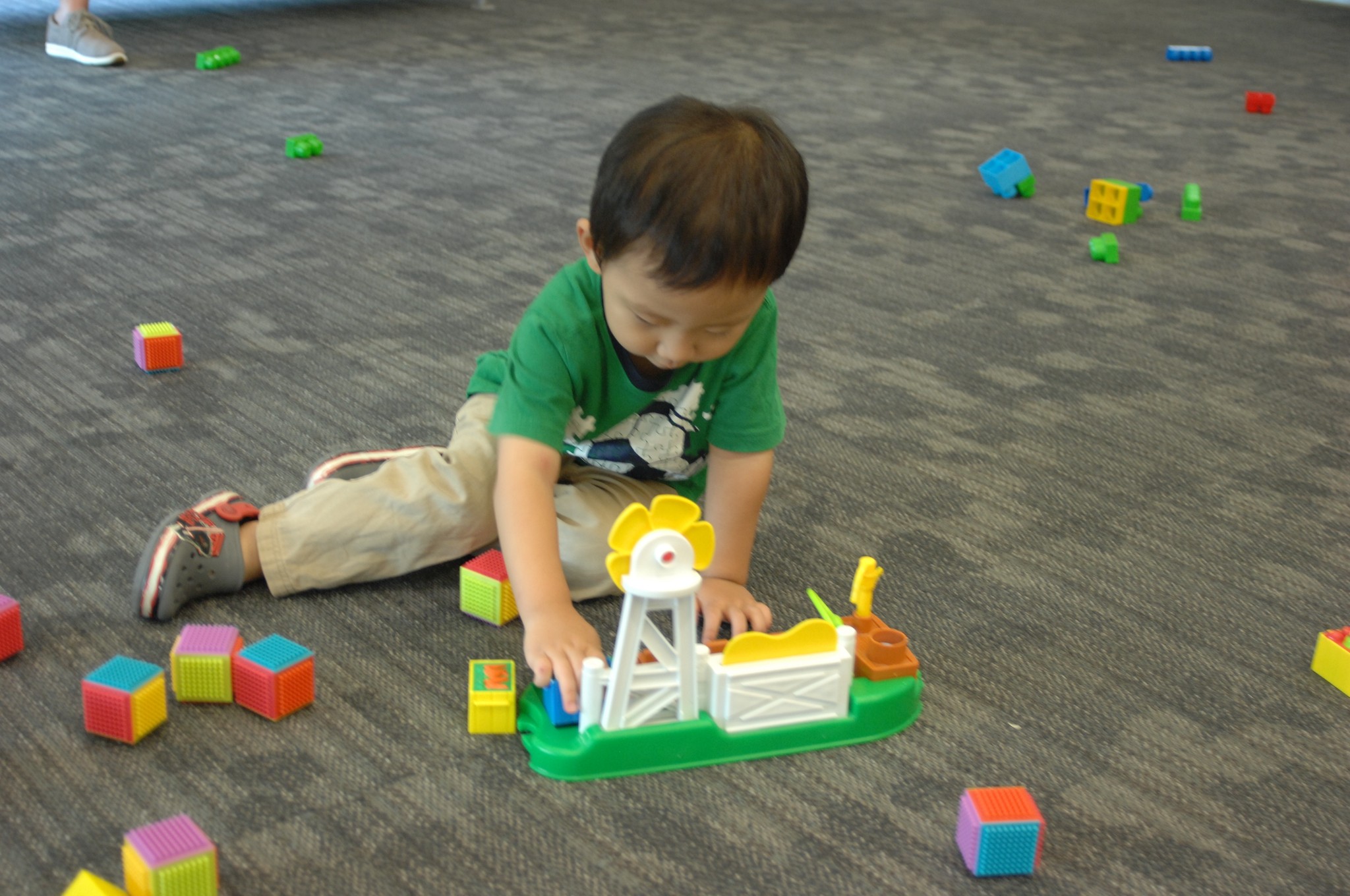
[133,491,260,622]
[306,446,444,489]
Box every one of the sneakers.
[45,9,127,65]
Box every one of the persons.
[44,1,128,66]
[134,96,809,712]
